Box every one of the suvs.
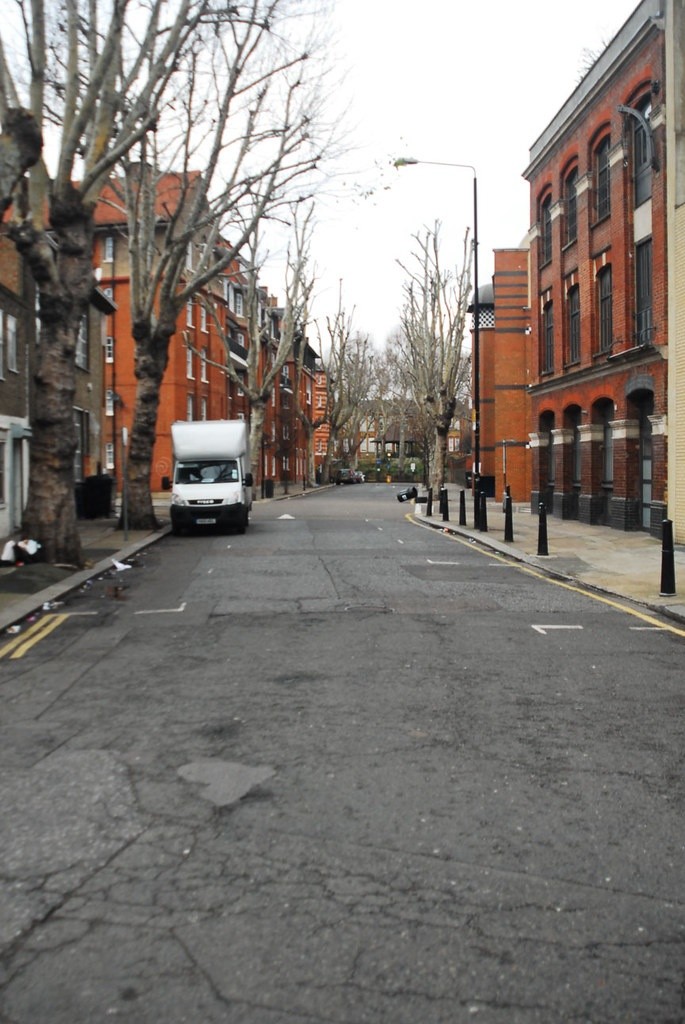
[336,468,357,485]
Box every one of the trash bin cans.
[396,486,418,503]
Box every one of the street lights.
[392,157,482,501]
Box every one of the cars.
[354,471,366,484]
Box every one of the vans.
[161,418,254,534]
